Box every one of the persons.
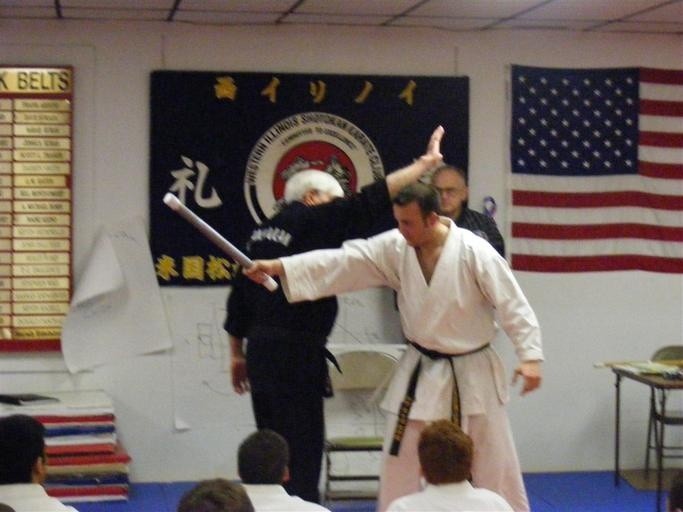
[395,165,506,313]
[0,414,83,512]
[241,182,544,512]
[235,430,330,511]
[223,124,444,511]
[176,477,254,511]
[386,419,517,511]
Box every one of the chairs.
[644,345,683,486]
[319,348,403,509]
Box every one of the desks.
[609,360,683,512]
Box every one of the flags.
[512,66,683,275]
